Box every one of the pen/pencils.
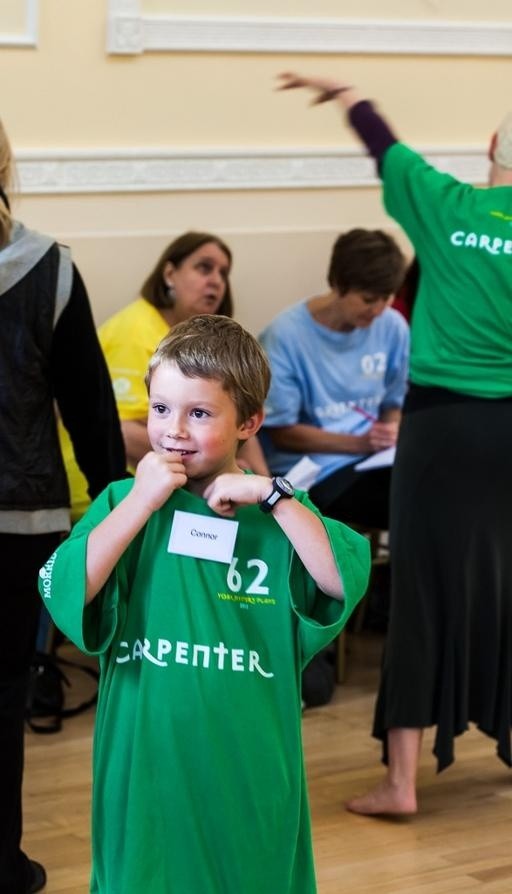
[351,404,377,427]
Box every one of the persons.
[52,231,275,525]
[258,225,411,536]
[2,116,130,894]
[34,309,376,894]
[273,65,512,821]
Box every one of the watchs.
[256,477,298,513]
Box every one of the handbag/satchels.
[20,645,104,736]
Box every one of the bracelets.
[311,80,349,102]
